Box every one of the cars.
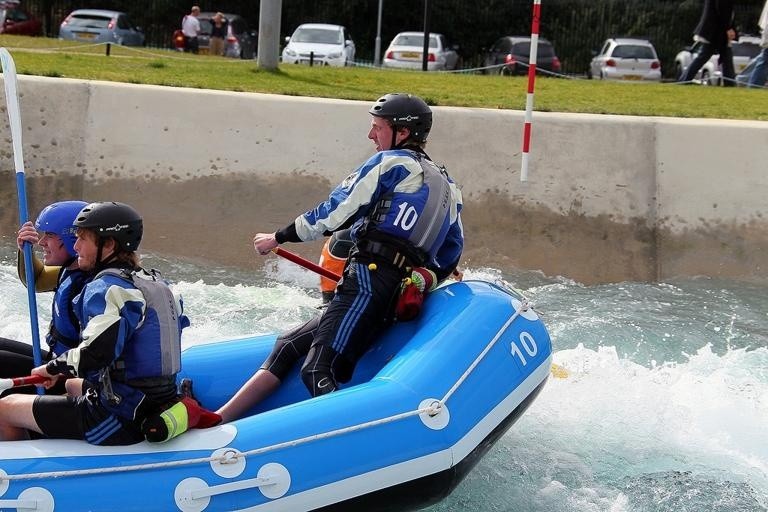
[476,34,564,78]
[381,30,462,72]
[0,0,45,37]
[279,22,358,67]
[585,34,666,84]
[57,6,147,48]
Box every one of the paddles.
[0,45,45,396]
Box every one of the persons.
[181,6,201,55]
[0,199,91,398]
[746,0,768,88]
[208,13,229,56]
[214,92,464,428]
[677,0,738,87]
[0,201,189,446]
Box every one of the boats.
[0,270,562,512]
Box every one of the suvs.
[670,30,763,87]
[172,10,258,58]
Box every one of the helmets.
[368,92,431,143]
[33,201,85,258]
[73,202,143,253]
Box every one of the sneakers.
[179,377,201,407]
